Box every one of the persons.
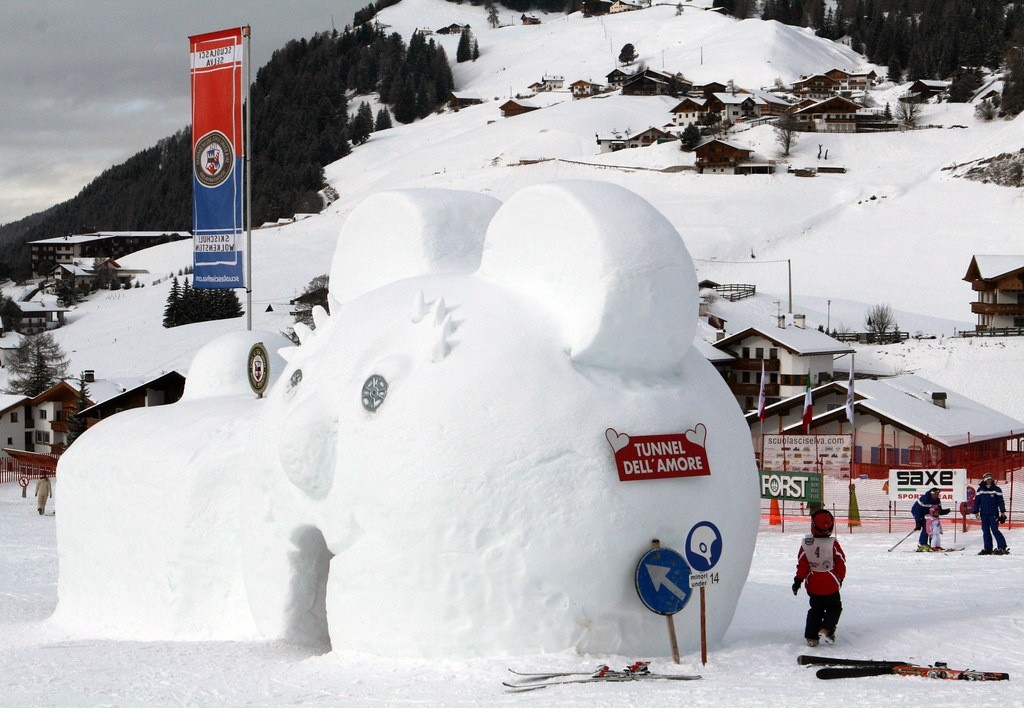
[35,470,52,515]
[911,488,951,552]
[973,473,1010,555]
[792,509,846,646]
[925,507,946,551]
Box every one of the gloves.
[944,508,950,514]
[839,579,843,588]
[998,515,1006,524]
[792,576,803,596]
[916,522,921,530]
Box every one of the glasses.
[811,510,834,533]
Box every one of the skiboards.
[797,655,908,680]
[501,660,702,687]
[918,544,966,553]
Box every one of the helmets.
[930,505,939,516]
[811,513,833,536]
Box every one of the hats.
[982,472,992,481]
[930,488,939,495]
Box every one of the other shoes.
[917,544,933,551]
[994,548,1006,554]
[978,549,992,556]
[931,547,945,551]
[819,628,835,645]
[807,638,819,647]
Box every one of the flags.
[846,355,854,425]
[802,371,812,434]
[189,26,245,289]
[758,360,766,419]
